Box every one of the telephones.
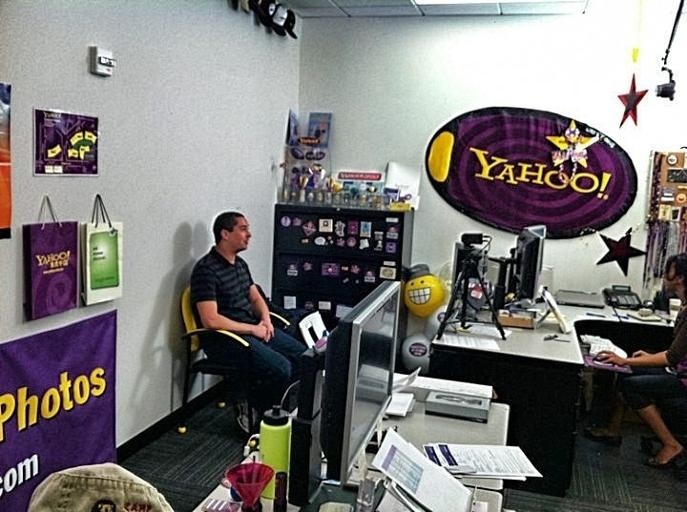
[602,285,641,311]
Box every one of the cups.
[224,463,274,511]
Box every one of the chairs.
[178,285,290,433]
[641,365,687,454]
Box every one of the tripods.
[435,261,507,343]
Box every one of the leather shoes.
[643,446,687,467]
[583,424,623,446]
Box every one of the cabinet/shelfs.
[272,202,414,332]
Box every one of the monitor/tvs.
[526,224,545,277]
[512,230,544,301]
[321,279,402,488]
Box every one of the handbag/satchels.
[22,196,78,320]
[80,196,123,306]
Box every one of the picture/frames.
[32,109,99,177]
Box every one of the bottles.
[259,405,290,499]
[272,471,287,512]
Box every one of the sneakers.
[237,401,256,433]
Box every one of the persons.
[593,253,687,466]
[190,210,309,440]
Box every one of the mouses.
[593,350,615,367]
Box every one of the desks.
[432,305,675,498]
[193,401,510,512]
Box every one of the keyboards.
[581,334,626,360]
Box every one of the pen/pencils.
[555,339,570,342]
[612,314,629,319]
[587,313,605,318]
